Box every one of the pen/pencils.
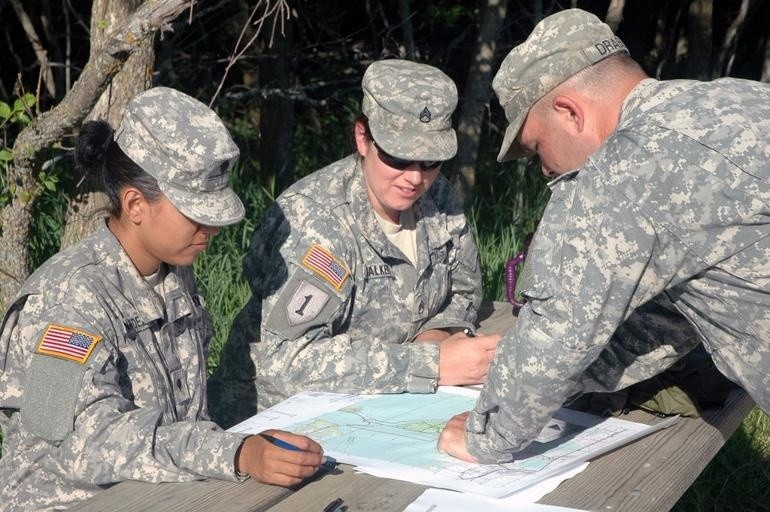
[323,499,348,512]
[464,329,474,337]
[257,433,335,469]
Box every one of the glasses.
[367,130,443,172]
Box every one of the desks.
[65,300,756,512]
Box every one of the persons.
[436,6,770,466]
[209,59,504,430]
[0,85,329,512]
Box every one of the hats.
[491,7,633,164]
[110,84,249,228]
[358,56,461,163]
